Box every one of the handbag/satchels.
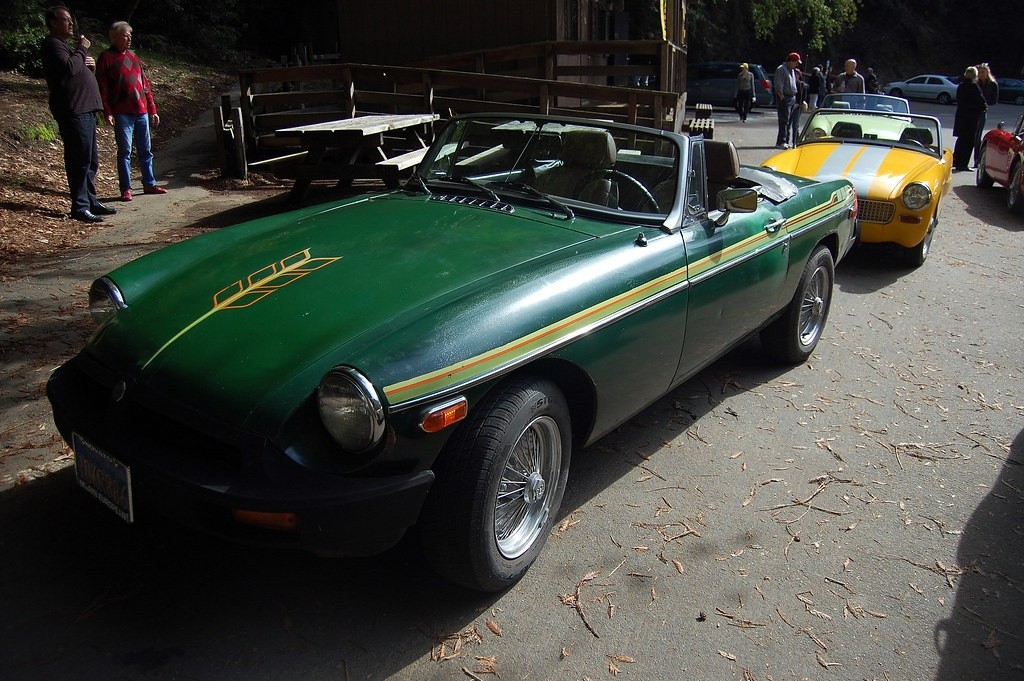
[802,101,808,112]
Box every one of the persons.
[953,67,988,172]
[735,63,756,123]
[41,6,117,222]
[95,21,167,200]
[809,65,833,108]
[866,68,879,94]
[787,68,810,145]
[774,54,802,149]
[836,59,865,108]
[973,63,997,168]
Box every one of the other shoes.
[777,143,788,149]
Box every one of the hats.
[814,67,820,71]
[789,52,802,63]
[740,63,748,68]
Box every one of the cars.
[977,113,1024,214]
[882,74,960,105]
[797,93,919,146]
[755,107,955,270]
[994,77,1024,106]
[41,108,863,596]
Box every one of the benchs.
[688,118,715,141]
[695,103,713,119]
[375,141,469,172]
[248,150,308,172]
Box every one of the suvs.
[626,60,775,113]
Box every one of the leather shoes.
[90,202,116,214]
[72,210,104,222]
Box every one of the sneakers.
[144,186,168,194]
[121,189,132,201]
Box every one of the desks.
[275,114,440,214]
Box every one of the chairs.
[899,127,935,153]
[875,104,894,112]
[831,122,863,138]
[829,101,851,109]
[535,130,618,208]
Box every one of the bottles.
[802,100,808,112]
